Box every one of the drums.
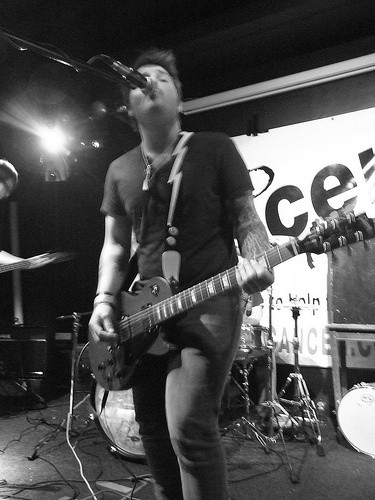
[93,380,151,464]
[231,322,274,362]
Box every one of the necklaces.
[140,142,177,191]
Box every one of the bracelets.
[94,291,112,296]
[93,295,116,308]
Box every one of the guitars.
[0,248,72,279]
[88,211,374,392]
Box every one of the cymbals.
[258,301,326,311]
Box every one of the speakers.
[0,324,55,380]
[325,219,375,333]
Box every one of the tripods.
[28,312,94,461]
[218,294,326,483]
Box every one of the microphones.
[100,55,156,95]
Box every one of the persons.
[0,159,56,270]
[88,49,275,500]
